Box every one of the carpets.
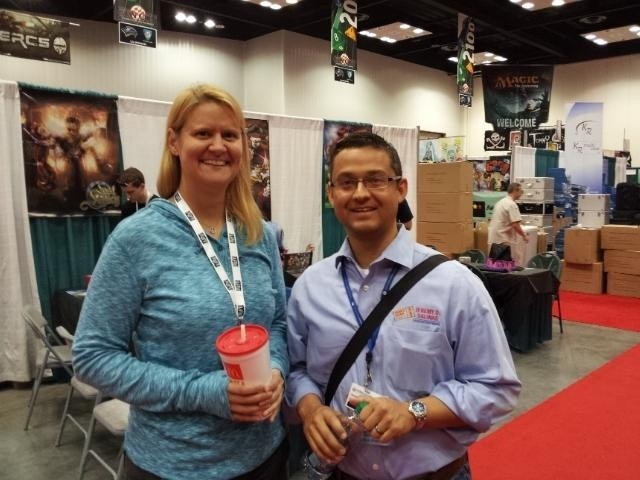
[550,287,639,334]
[467,342,640,479]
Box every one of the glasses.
[329,175,404,190]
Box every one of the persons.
[285,131,524,479]
[394,197,415,232]
[486,182,530,267]
[66,80,293,477]
[20,109,118,216]
[118,167,161,219]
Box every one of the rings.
[374,424,385,436]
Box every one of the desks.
[470,265,555,353]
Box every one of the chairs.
[525,251,564,334]
[17,312,135,480]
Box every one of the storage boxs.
[418,157,640,297]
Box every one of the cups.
[458,256,472,266]
[215,324,276,421]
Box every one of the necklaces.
[198,215,224,237]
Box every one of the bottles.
[300,400,370,477]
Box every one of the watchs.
[406,398,429,433]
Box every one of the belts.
[424,452,469,479]
